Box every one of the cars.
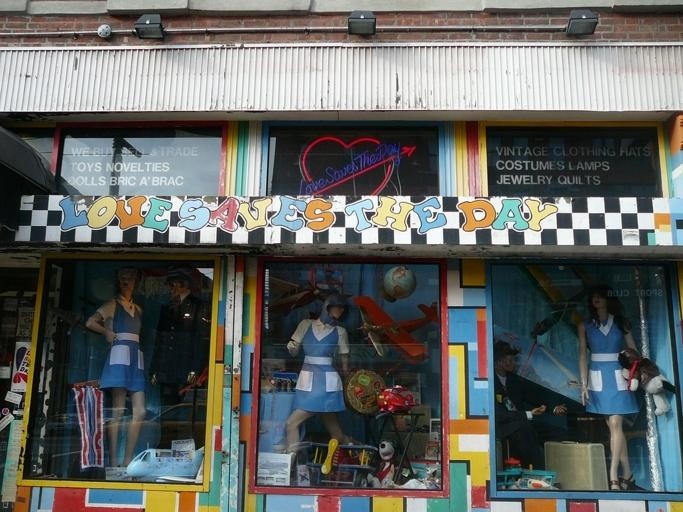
[48,386,207,460]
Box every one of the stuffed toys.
[364,436,407,492]
[614,346,676,415]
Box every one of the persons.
[494,339,569,470]
[284,293,353,453]
[150,268,206,447]
[579,285,647,491]
[85,265,148,469]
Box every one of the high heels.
[610,474,635,491]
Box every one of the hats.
[494,341,520,361]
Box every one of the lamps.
[566,9,598,35]
[348,11,376,35]
[134,14,164,39]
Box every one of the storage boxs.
[380,404,432,468]
